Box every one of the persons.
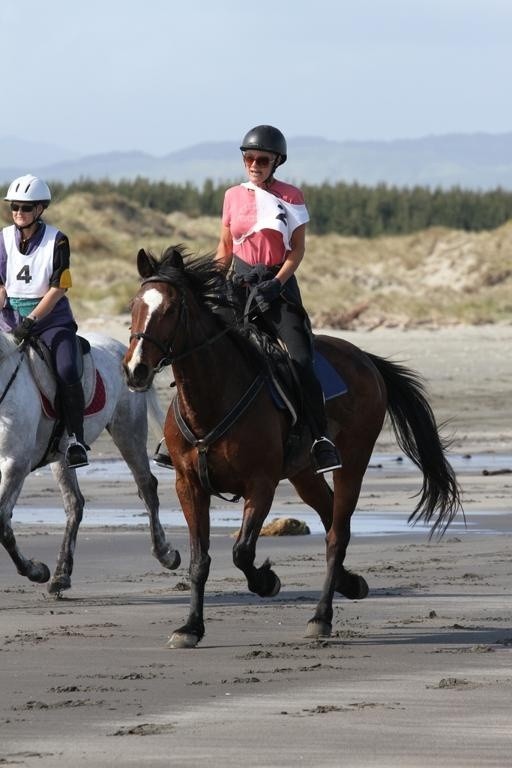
[0,172,91,468]
[153,124,342,471]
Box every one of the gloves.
[10,318,36,352]
[253,277,284,313]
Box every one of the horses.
[0,330,182,594]
[119,244,467,651]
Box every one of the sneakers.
[66,443,87,464]
[313,447,342,473]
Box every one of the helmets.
[240,124,287,166]
[4,174,52,202]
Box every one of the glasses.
[242,153,276,167]
[11,203,37,213]
